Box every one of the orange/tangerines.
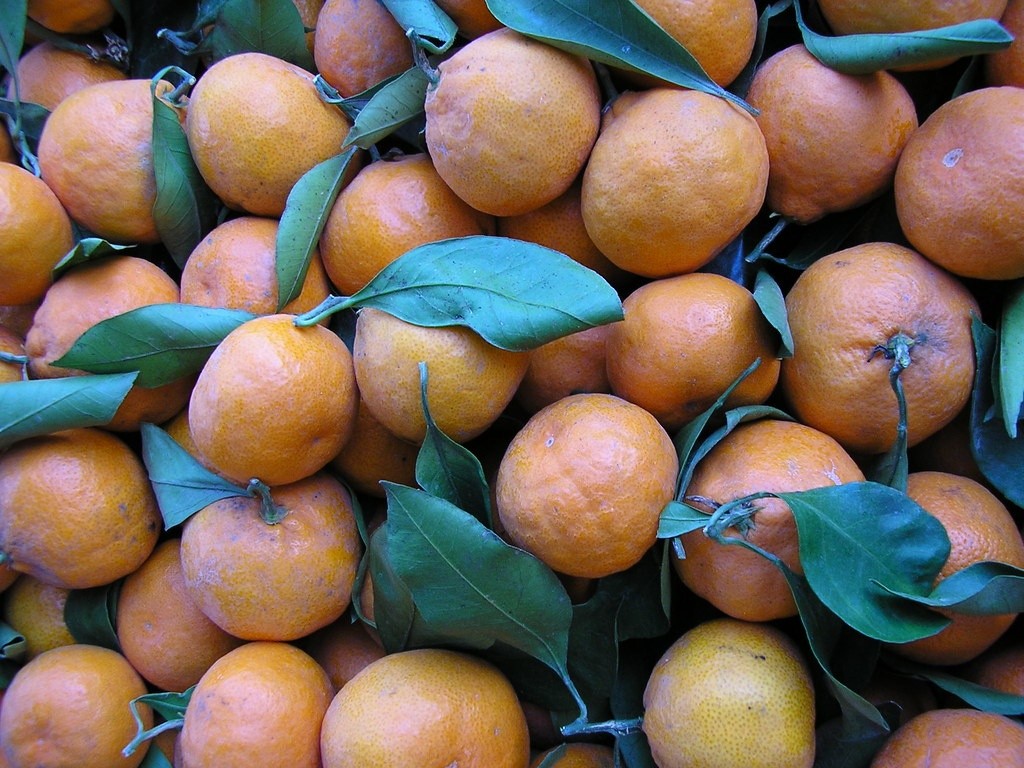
[0,0,1022,767]
